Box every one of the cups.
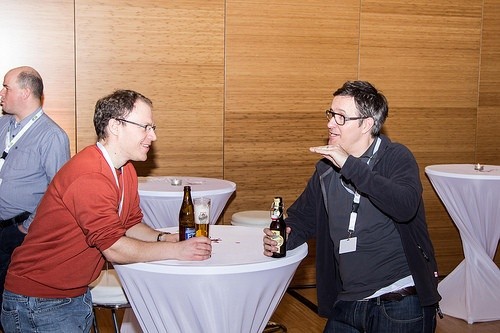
[193,199,210,237]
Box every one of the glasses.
[325,110,370,126]
[115,117,156,133]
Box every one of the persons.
[261,79,445,333]
[0,65,72,333]
[0,89,212,333]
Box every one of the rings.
[327,145,329,149]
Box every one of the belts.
[0,213,29,231]
[371,287,416,303]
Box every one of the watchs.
[156,231,171,241]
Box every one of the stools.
[231,210,287,227]
[89,269,132,333]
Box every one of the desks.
[425,164,500,325]
[110,224,309,333]
[138,176,237,229]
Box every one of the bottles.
[270,197,286,258]
[179,186,195,241]
